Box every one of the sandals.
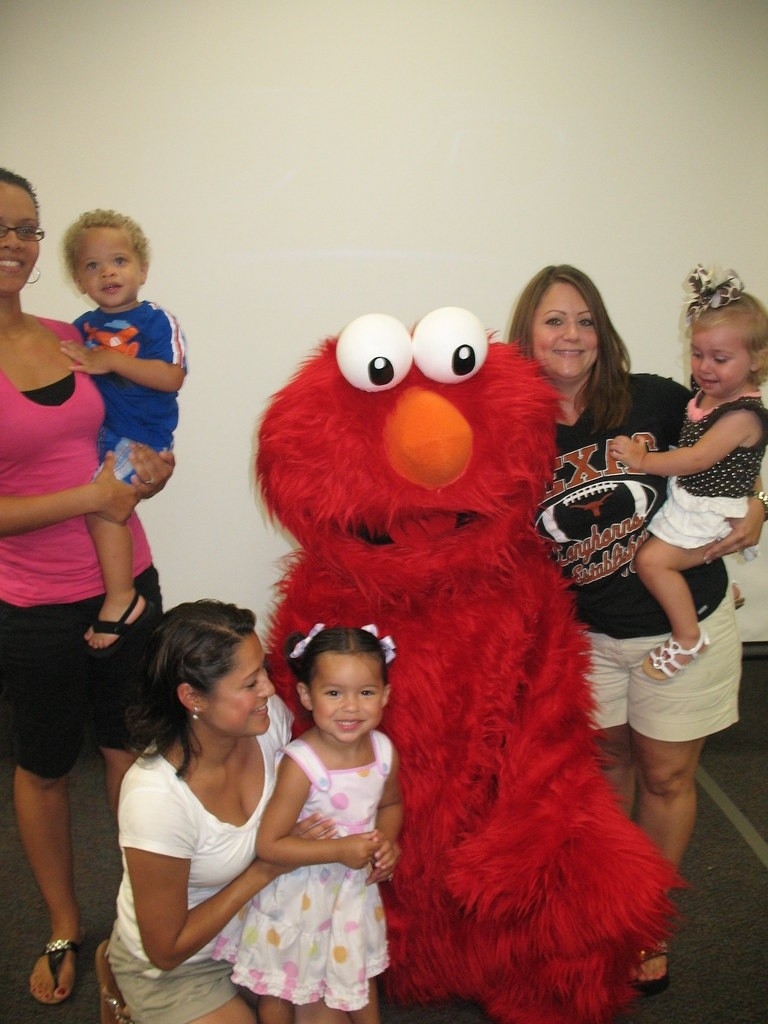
[731,580,744,610]
[629,945,670,996]
[86,591,154,658]
[643,627,710,681]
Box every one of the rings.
[388,874,393,880]
[144,479,152,484]
[736,548,743,552]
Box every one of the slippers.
[95,940,131,1024]
[29,927,86,1004]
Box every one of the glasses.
[0,224,45,241]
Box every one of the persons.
[0,167,176,1024]
[507,264,768,997]
[58,210,189,651]
[229,623,403,1024]
[605,265,768,683]
[105,600,404,1024]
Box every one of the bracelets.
[750,490,768,522]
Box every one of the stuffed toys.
[253,308,684,1024]
